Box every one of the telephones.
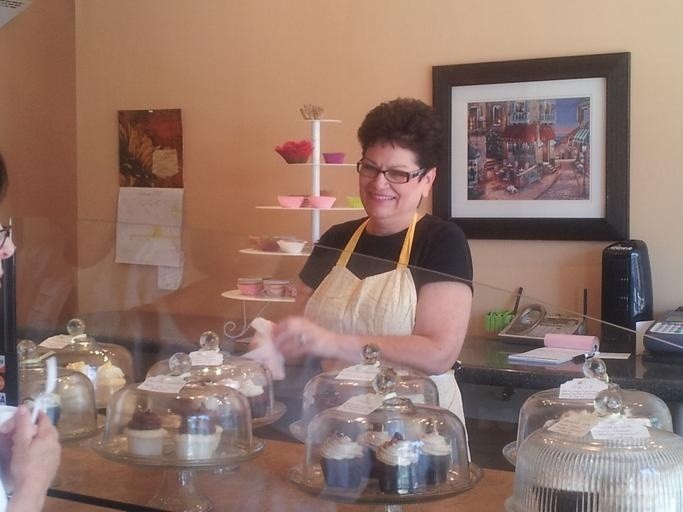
[498,287,588,344]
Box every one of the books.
[508,333,600,364]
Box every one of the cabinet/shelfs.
[218,115,367,340]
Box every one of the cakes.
[374,432,421,493]
[124,409,167,455]
[319,428,362,488]
[66,361,98,401]
[600,468,677,511]
[533,471,599,511]
[171,415,223,460]
[414,417,453,483]
[93,361,126,403]
[354,422,392,479]
[173,378,268,418]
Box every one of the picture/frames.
[427,49,634,247]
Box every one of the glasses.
[355,155,430,185]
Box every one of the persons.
[0,158,62,511]
[250,97,474,460]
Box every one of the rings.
[295,330,304,345]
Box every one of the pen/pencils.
[573,351,600,363]
[32,396,41,426]
